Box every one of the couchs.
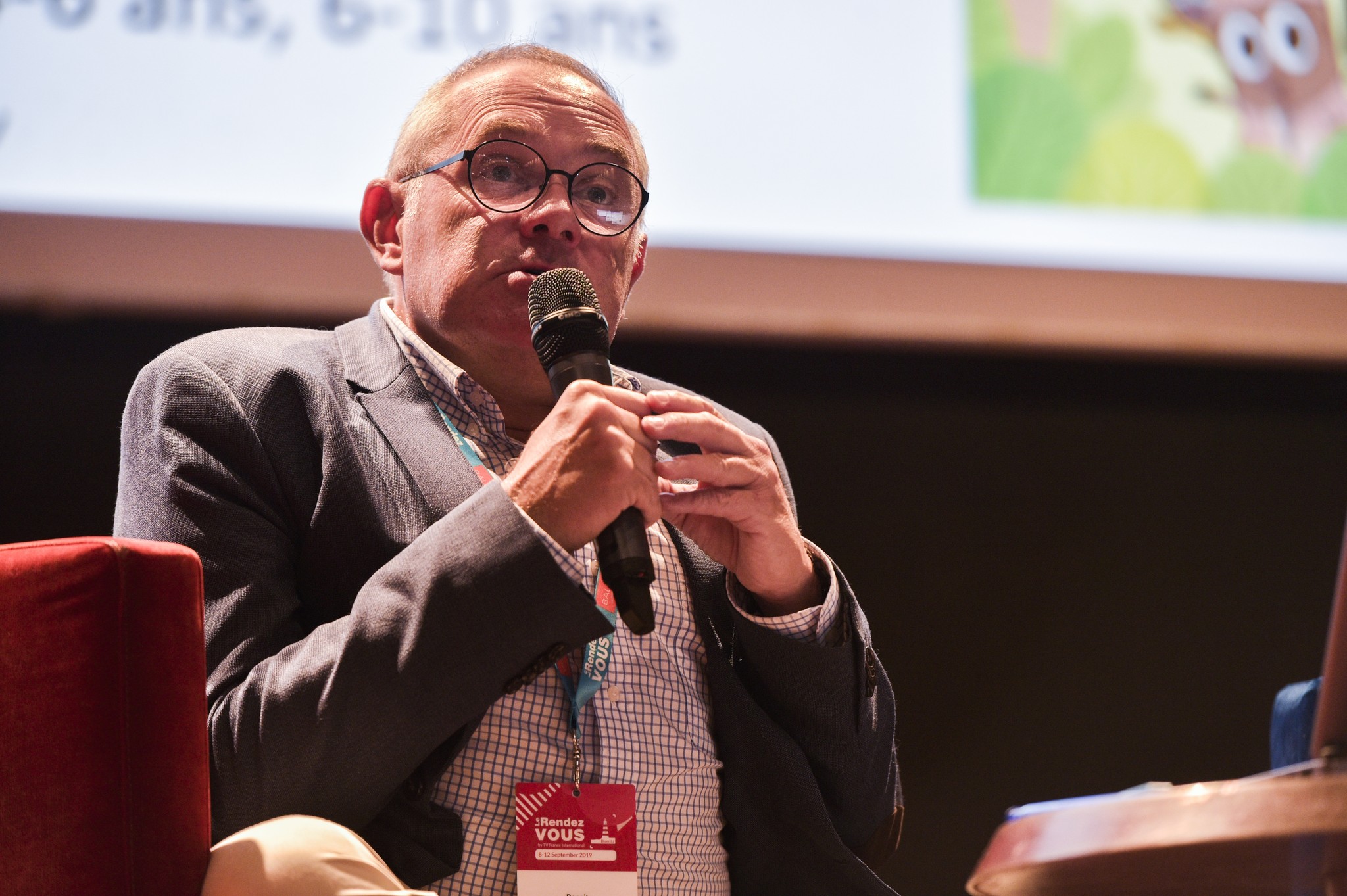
[2,540,213,892]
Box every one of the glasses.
[398,139,650,237]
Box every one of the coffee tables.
[967,767,1347,894]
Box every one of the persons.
[113,42,906,895]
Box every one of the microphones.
[528,269,662,635]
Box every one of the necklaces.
[505,422,536,432]
